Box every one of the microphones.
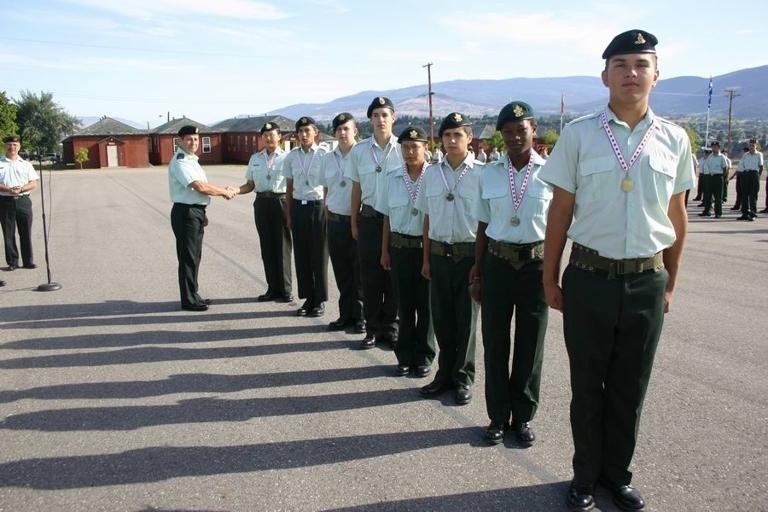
[45,153,62,157]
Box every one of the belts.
[361,204,384,219]
[325,204,352,224]
[0,194,30,200]
[293,199,322,206]
[743,170,758,173]
[256,191,286,200]
[568,241,664,275]
[174,203,207,208]
[429,239,477,259]
[486,236,545,263]
[388,232,424,250]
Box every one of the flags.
[558,95,566,117]
[708,77,714,109]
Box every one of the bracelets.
[21,187,24,193]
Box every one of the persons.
[469,146,500,163]
[321,112,366,335]
[425,143,443,164]
[226,122,297,302]
[533,145,552,158]
[380,126,437,376]
[413,112,479,405]
[0,135,40,271]
[536,28,697,511]
[469,100,551,442]
[345,96,399,351]
[693,137,768,218]
[166,125,236,311]
[283,116,329,317]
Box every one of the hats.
[177,125,199,137]
[2,137,17,143]
[260,121,279,136]
[366,96,395,118]
[602,29,659,59]
[438,112,472,137]
[705,139,758,153]
[397,126,428,144]
[332,112,353,134]
[295,116,315,133]
[495,100,536,131]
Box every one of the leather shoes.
[7,264,38,271]
[413,357,432,376]
[181,304,209,311]
[610,483,646,512]
[258,289,398,350]
[566,476,598,512]
[706,173,723,177]
[453,382,473,404]
[510,421,536,446]
[203,298,215,304]
[417,378,454,399]
[693,196,768,221]
[485,419,510,445]
[395,363,411,376]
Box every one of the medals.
[410,206,419,217]
[511,216,521,228]
[376,167,383,172]
[304,178,309,187]
[266,174,271,180]
[339,180,347,187]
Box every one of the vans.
[40,153,60,161]
